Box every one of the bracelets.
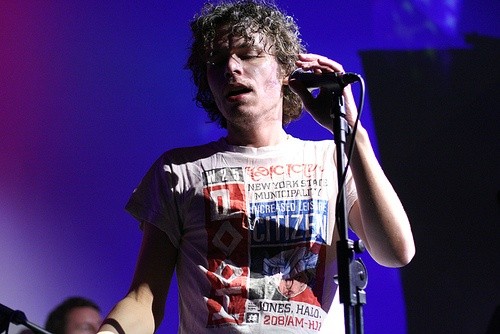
[97,318,125,334]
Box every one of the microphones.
[287,66,360,96]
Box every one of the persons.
[44,296,105,334]
[92,0,416,334]
